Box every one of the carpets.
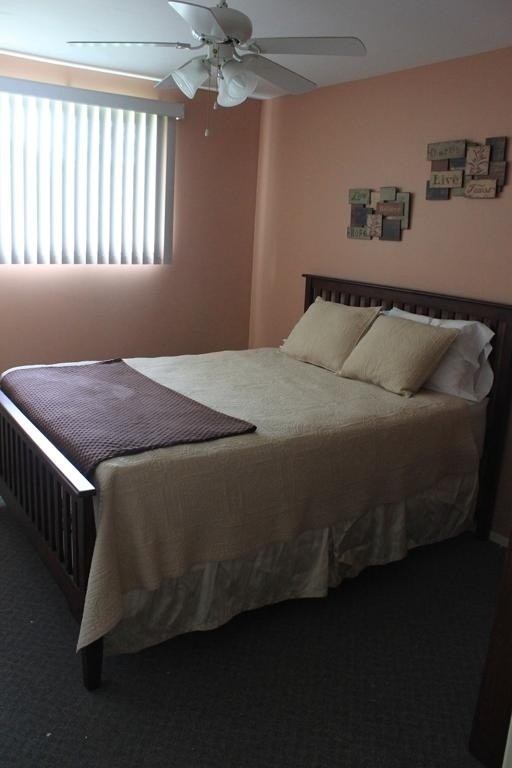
[0,500,507,768]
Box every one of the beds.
[0,273,512,692]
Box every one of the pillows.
[337,314,460,399]
[389,304,495,403]
[277,296,383,372]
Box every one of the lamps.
[66,1,368,138]
